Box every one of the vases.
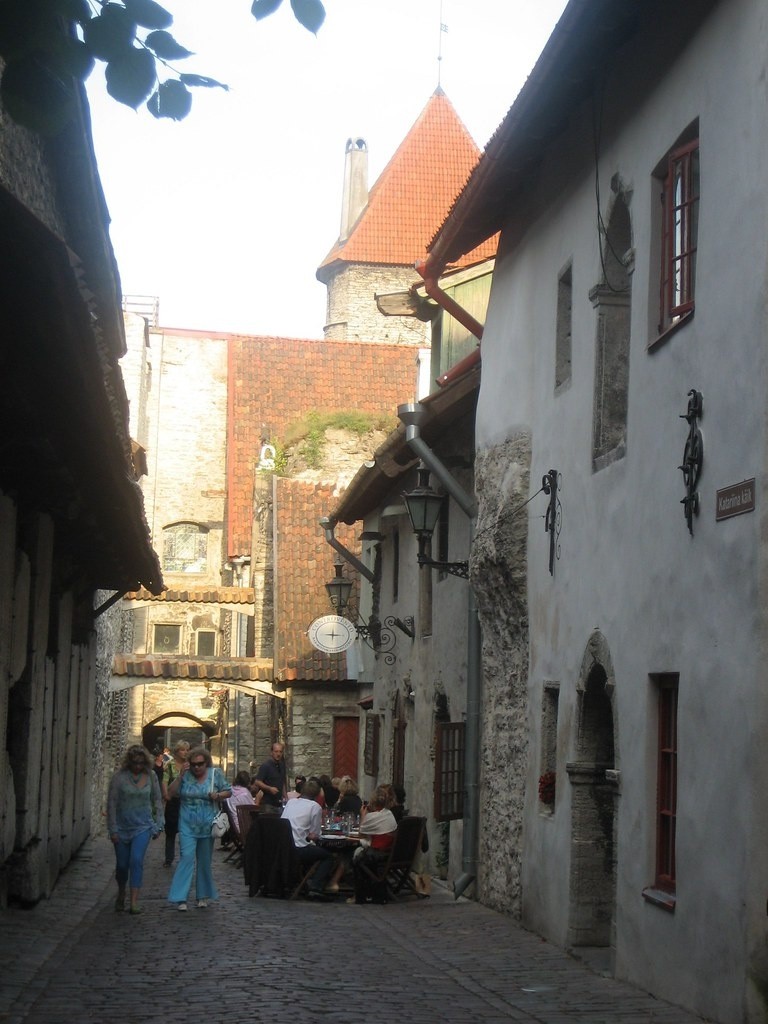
[548,802,555,814]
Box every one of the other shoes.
[346,898,355,905]
[178,902,187,911]
[163,860,171,868]
[116,899,123,910]
[324,884,340,892]
[198,898,208,907]
[131,906,141,913]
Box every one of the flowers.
[539,769,556,805]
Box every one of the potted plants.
[433,824,449,881]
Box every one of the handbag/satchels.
[152,810,157,823]
[211,813,230,838]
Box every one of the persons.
[167,745,232,912]
[107,745,165,915]
[281,773,406,903]
[254,743,289,814]
[226,771,255,835]
[152,747,174,785]
[161,739,190,868]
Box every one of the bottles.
[329,811,335,832]
[355,815,360,824]
[346,816,352,837]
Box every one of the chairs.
[217,798,430,903]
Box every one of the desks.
[307,828,360,901]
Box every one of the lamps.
[325,552,416,664]
[399,460,469,581]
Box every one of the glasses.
[191,759,207,767]
